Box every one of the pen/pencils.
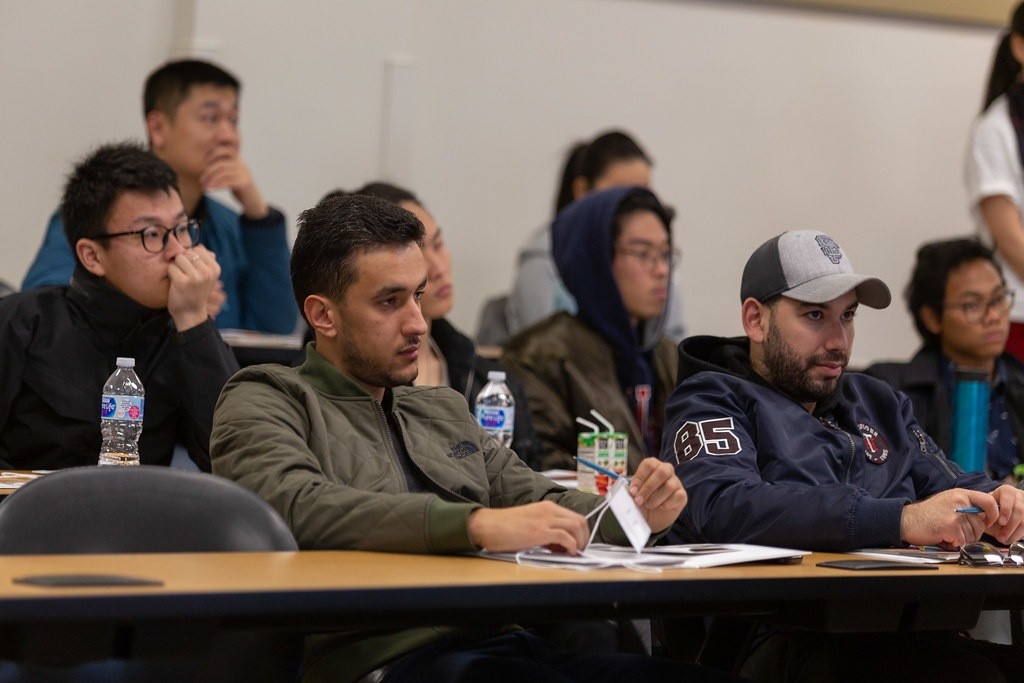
[954,505,1000,513]
[575,456,629,483]
[12,573,172,587]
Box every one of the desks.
[0,323,1024,683]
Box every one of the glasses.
[958,541,1024,566]
[87,220,200,254]
[618,246,683,271]
[934,288,1016,323]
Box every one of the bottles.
[97,356,145,465]
[474,370,515,450]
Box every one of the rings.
[191,256,199,263]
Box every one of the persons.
[964,0,1024,362]
[859,238,1024,491]
[0,142,240,472]
[24,57,298,334]
[660,229,1024,653]
[510,132,685,342]
[207,193,753,683]
[497,184,678,474]
[357,182,542,471]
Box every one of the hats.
[739,230,891,310]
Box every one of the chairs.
[0,463,307,683]
